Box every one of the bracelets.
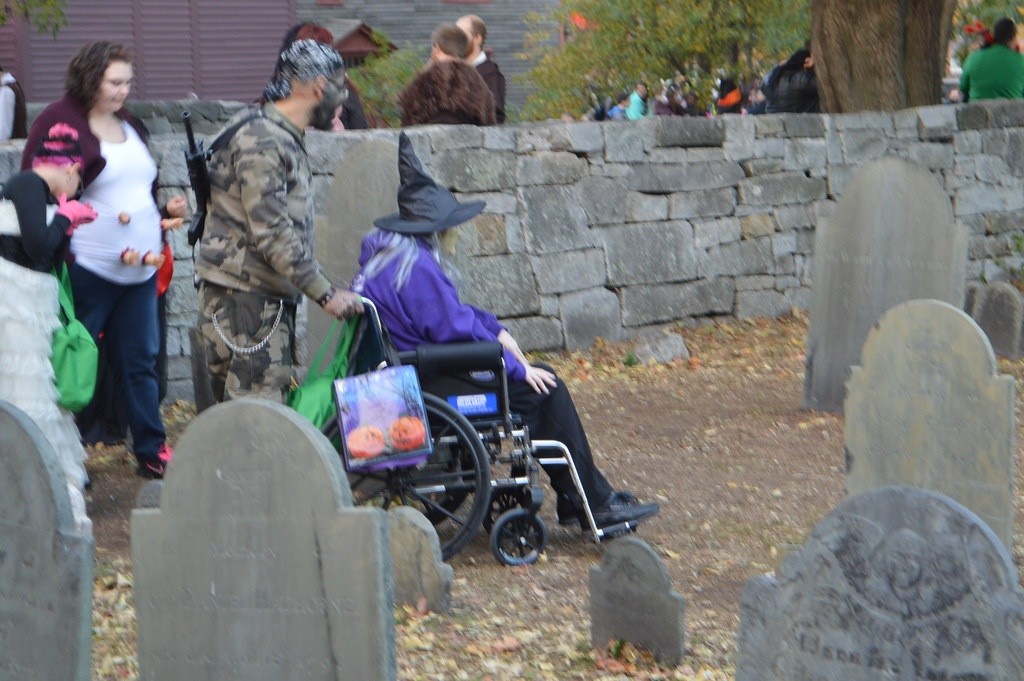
[315,286,336,308]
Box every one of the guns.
[180,110,212,246]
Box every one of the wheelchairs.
[321,297,638,568]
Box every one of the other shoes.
[555,492,659,539]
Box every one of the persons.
[188,39,364,416]
[279,21,369,132]
[944,88,962,105]
[397,14,506,127]
[958,16,1024,103]
[0,65,27,139]
[0,39,187,494]
[350,130,660,537]
[608,39,822,121]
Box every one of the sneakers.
[136,456,168,481]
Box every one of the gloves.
[55,193,98,236]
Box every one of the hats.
[375,131,487,233]
[31,123,83,171]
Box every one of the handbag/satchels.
[290,314,358,453]
[334,296,433,473]
[51,257,97,409]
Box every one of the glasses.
[331,76,352,97]
